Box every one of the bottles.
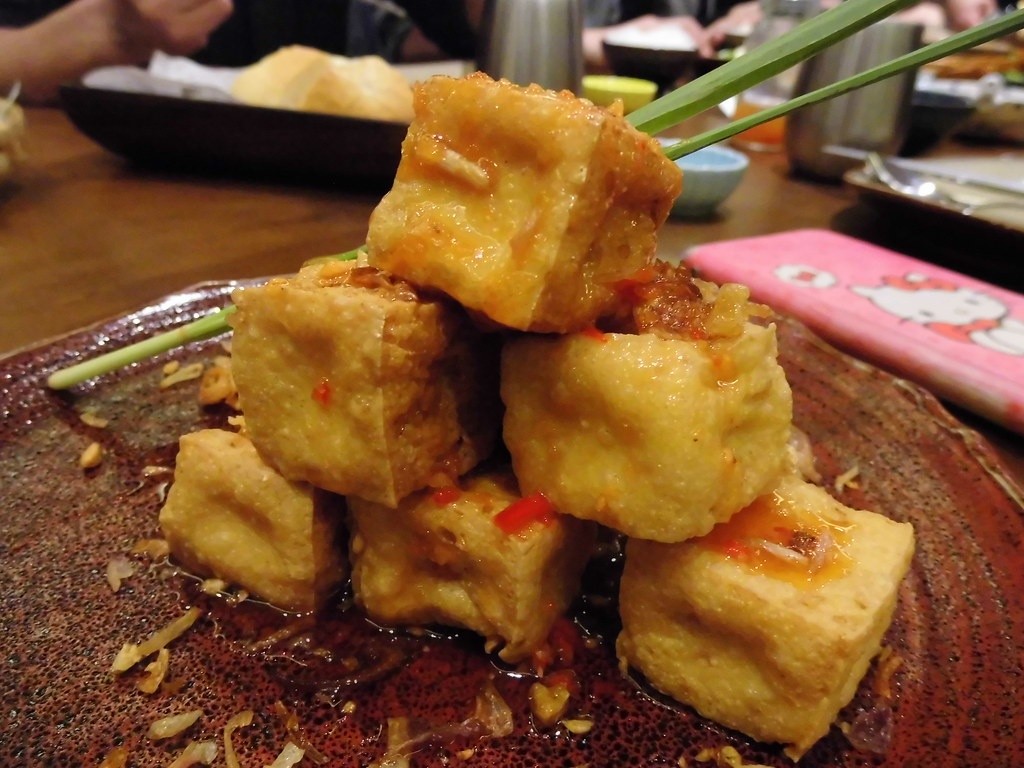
[480,1,584,100]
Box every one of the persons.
[1,1,1024,107]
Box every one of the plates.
[841,147,1024,253]
[61,81,416,196]
[0,263,1023,768]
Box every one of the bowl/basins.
[602,41,696,96]
[581,73,658,117]
[648,137,751,219]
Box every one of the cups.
[781,23,927,182]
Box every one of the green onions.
[46,0,1024,391]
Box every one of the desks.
[1,82,1024,500]
